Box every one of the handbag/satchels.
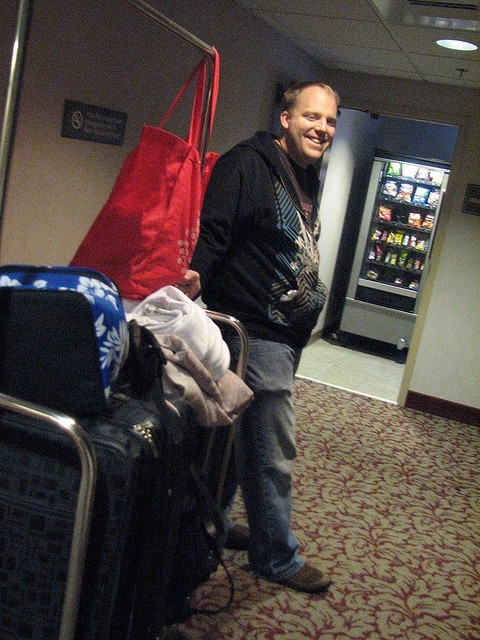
[69,47,221,298]
[0,265,129,412]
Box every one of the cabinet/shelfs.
[337,151,451,351]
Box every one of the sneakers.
[227,523,248,548]
[282,567,330,592]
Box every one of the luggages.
[1,395,196,640]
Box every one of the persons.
[174,79,341,593]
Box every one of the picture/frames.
[461,182,480,217]
